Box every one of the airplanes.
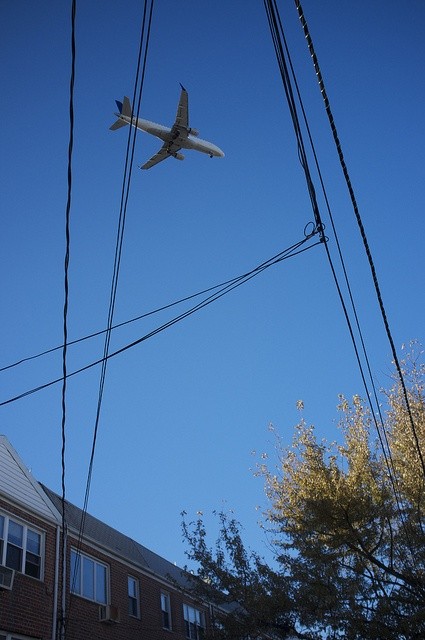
[106,81,226,169]
